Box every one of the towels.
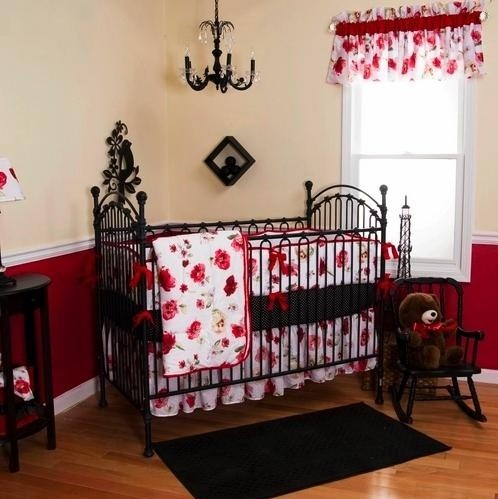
[153,229,252,377]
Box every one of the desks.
[0,271,57,473]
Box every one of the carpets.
[153,402,451,499]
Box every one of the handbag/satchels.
[0,362,40,443]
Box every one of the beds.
[91,181,388,459]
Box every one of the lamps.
[177,0,262,93]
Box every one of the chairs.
[396,279,487,425]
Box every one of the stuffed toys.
[399,291,464,368]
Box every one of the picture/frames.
[205,137,254,186]
